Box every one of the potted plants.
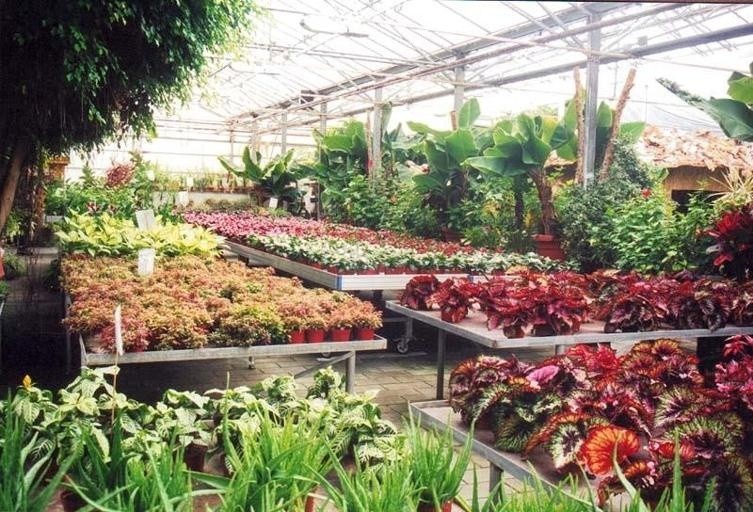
[0,72,752,512]
